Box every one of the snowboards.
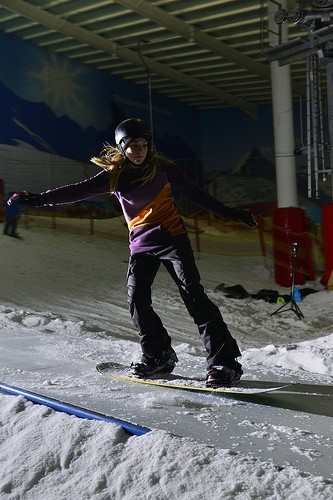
[96,362,295,395]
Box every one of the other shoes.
[131,356,175,379]
[206,362,243,388]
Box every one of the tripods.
[270,252,304,321]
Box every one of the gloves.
[231,208,258,228]
[7,190,37,206]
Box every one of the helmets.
[115,117,151,145]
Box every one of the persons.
[7,118,257,388]
[4,204,22,236]
